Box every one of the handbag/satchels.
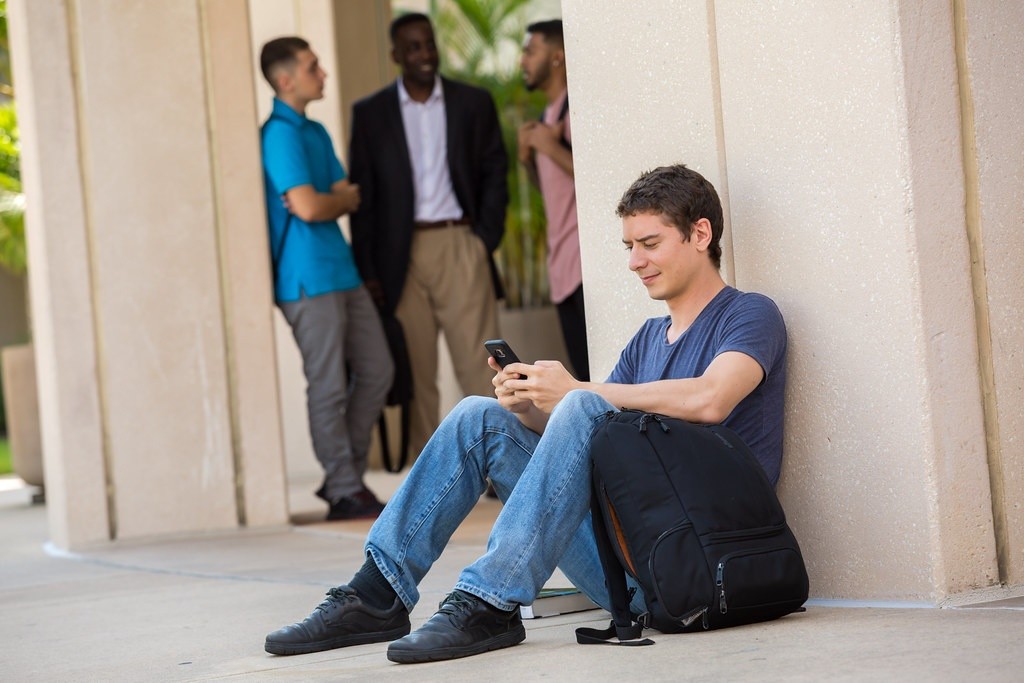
[371,309,415,407]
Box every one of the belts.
[415,220,469,228]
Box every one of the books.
[520,588,605,619]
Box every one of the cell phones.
[484,340,528,380]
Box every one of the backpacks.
[572,407,810,646]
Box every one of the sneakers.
[387,591,526,663]
[315,482,386,518]
[265,585,412,655]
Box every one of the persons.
[515,17,589,386]
[344,12,511,497]
[264,164,788,663]
[258,36,396,524]
